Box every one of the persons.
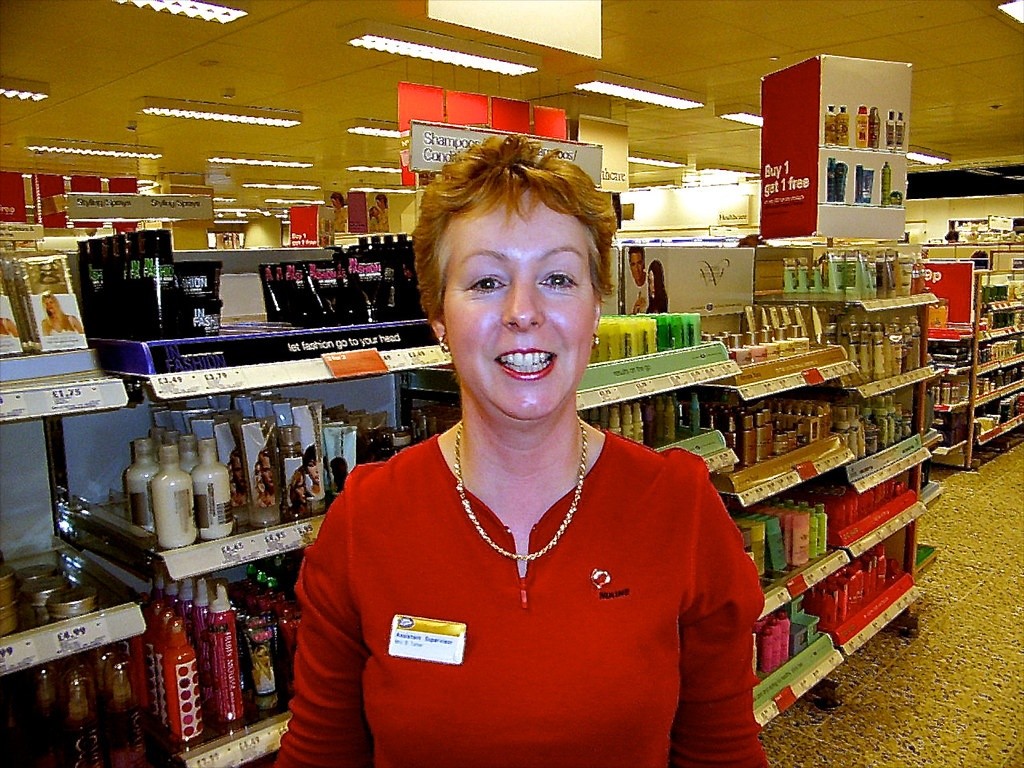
[945,230,960,242]
[623,246,669,314]
[274,134,771,768]
[330,193,390,232]
[0,292,83,339]
[225,444,350,528]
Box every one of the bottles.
[35,644,147,768]
[123,426,234,548]
[825,105,906,206]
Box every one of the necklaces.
[455,418,588,561]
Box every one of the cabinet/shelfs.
[0,237,1024,768]
